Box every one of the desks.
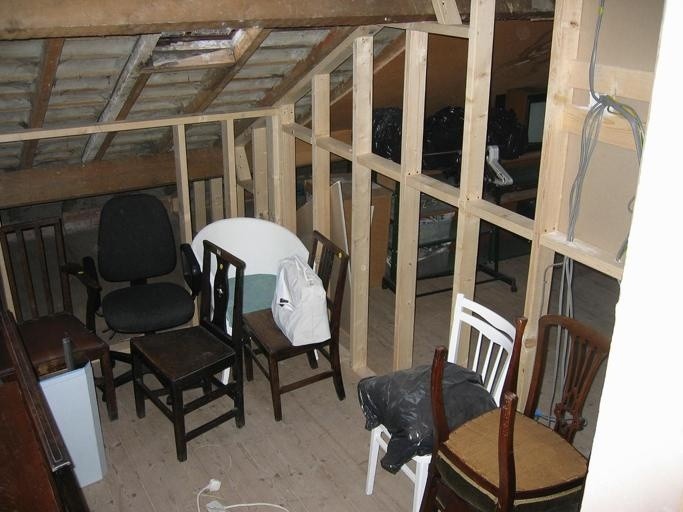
[2,381,86,510]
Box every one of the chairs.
[61,190,214,409]
[523,313,612,448]
[364,297,514,512]
[129,241,247,467]
[419,346,588,511]
[242,229,350,423]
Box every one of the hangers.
[457,144,513,188]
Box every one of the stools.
[2,311,120,424]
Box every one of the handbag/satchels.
[271,254,332,348]
